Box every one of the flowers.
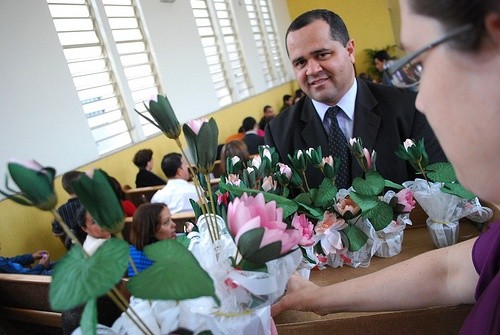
[0,93,478,335]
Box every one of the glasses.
[383,23,475,92]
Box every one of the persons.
[265,9,451,227]
[0,50,422,275]
[270,0,500,335]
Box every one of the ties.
[324,105,354,190]
